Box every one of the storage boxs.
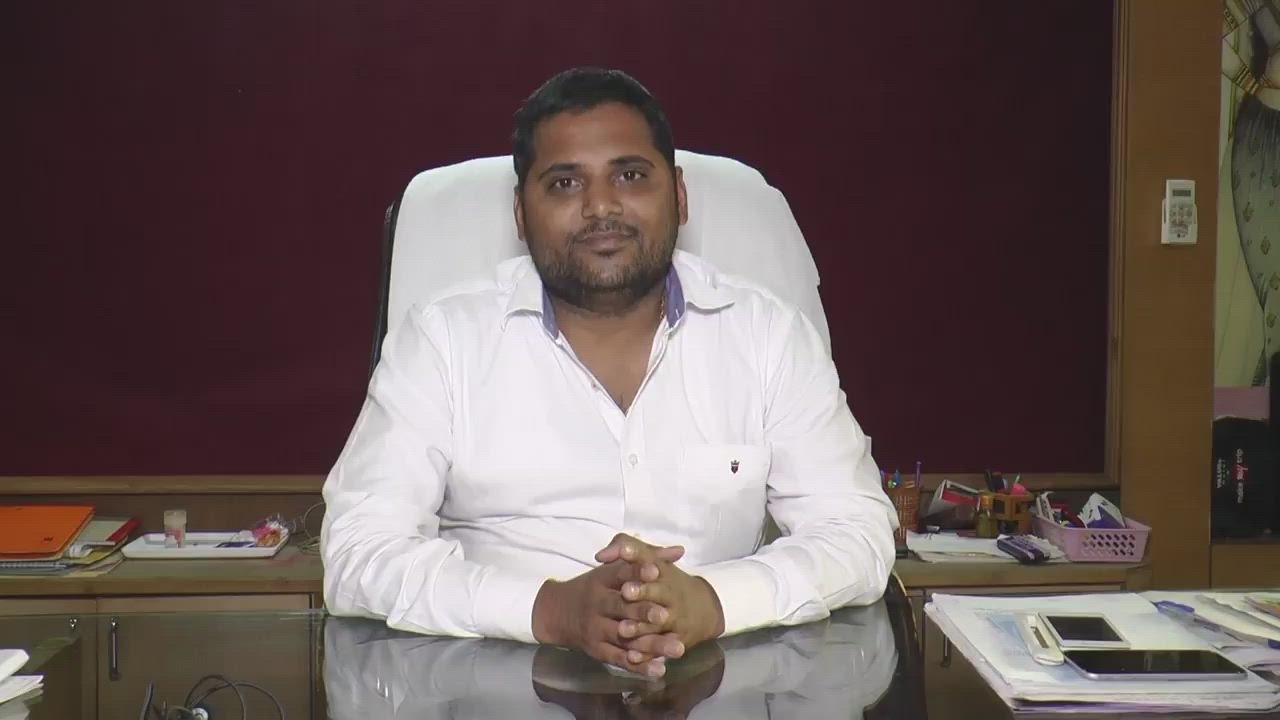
[928,478,981,515]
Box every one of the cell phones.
[1063,648,1249,682]
[1039,610,1129,652]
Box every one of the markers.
[982,468,1028,494]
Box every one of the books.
[0,502,142,576]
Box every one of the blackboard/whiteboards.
[0,0,1130,494]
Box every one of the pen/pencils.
[915,461,921,489]
[1154,600,1280,649]
[880,464,901,489]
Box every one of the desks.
[0,595,1280,720]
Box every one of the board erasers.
[998,536,1050,563]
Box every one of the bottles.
[976,496,998,538]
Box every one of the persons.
[318,64,900,680]
[322,615,899,720]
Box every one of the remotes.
[996,535,1051,561]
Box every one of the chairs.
[368,155,922,720]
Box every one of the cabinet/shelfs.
[926,585,1123,720]
[0,596,311,720]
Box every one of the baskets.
[1032,507,1152,562]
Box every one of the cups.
[164,509,187,548]
[884,488,920,547]
